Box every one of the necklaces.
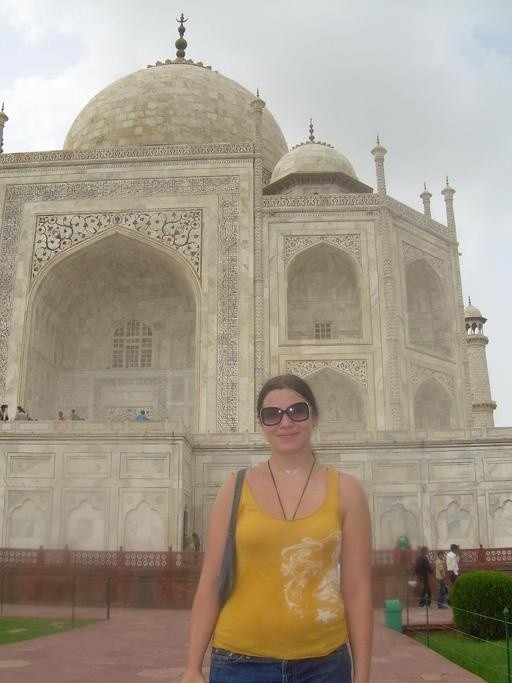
[268,456,318,520]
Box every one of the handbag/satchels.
[214,465,247,610]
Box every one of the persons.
[1,405,33,419]
[416,544,460,608]
[181,374,373,683]
[58,409,81,420]
[136,410,149,419]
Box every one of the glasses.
[259,400,311,426]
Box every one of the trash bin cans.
[385,599,401,632]
